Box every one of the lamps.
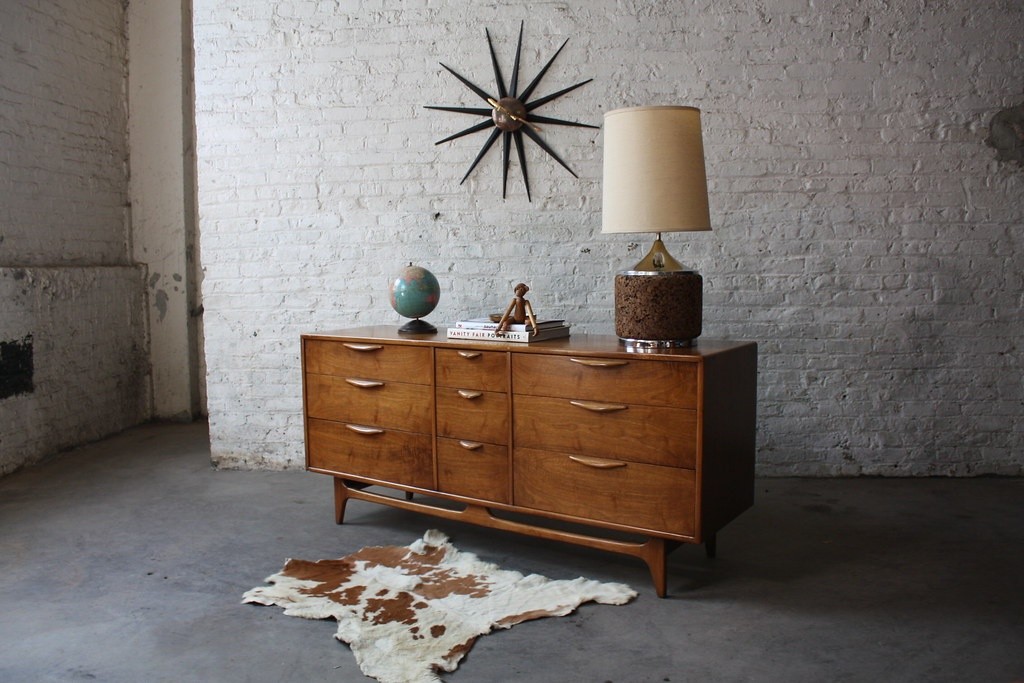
[600,106,712,348]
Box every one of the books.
[446,317,571,344]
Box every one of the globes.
[388,261,441,334]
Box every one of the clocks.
[422,18,599,202]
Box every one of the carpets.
[241,529,639,683]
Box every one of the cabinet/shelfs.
[299,325,758,599]
